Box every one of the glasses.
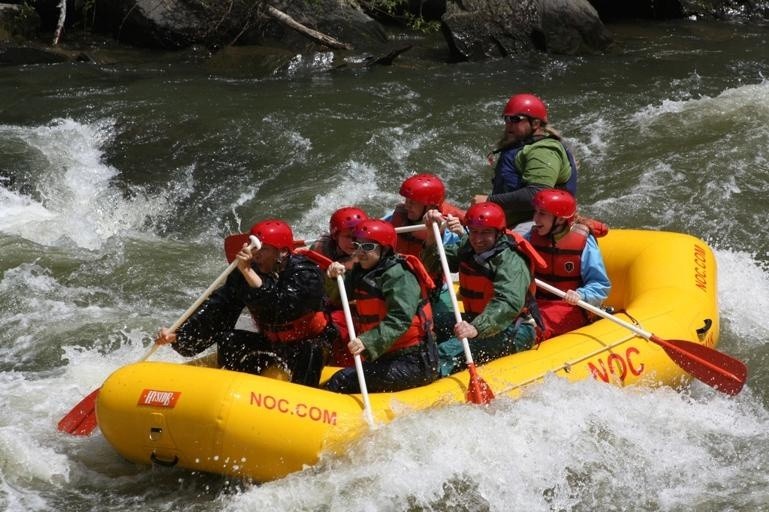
[504,115,528,122]
[351,240,378,252]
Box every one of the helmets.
[501,94,548,123]
[250,219,294,253]
[352,219,397,253]
[463,202,506,230]
[532,190,577,219]
[399,174,445,206]
[329,207,368,237]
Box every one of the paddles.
[57,234,262,438]
[431,220,496,405]
[533,277,748,396]
[224,212,454,266]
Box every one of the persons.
[153,221,326,387]
[389,173,466,261]
[470,93,576,232]
[323,219,439,394]
[525,189,611,345]
[309,207,368,299]
[423,202,537,377]
